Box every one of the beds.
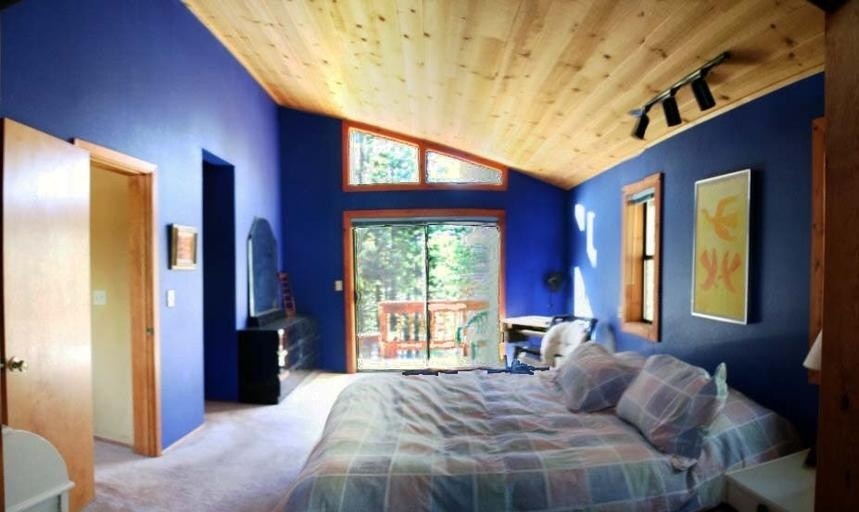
[275,340,798,512]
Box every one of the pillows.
[546,340,729,474]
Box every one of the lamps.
[630,51,726,140]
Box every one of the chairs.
[511,316,597,375]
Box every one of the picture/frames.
[618,172,662,339]
[688,168,752,325]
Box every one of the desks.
[498,309,559,364]
[238,314,321,407]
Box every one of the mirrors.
[247,216,281,317]
